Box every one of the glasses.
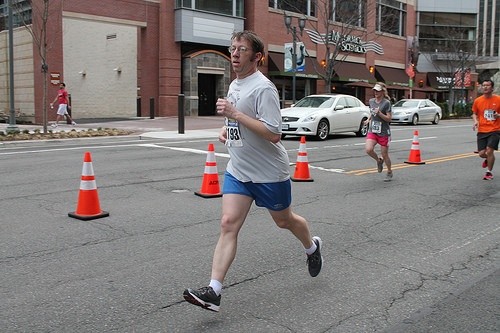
[228,46,255,55]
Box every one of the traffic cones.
[404,129,427,165]
[290,134,315,182]
[66,151,111,222]
[193,143,224,199]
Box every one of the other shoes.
[385,173,393,182]
[377,158,383,172]
[51,123,57,128]
[72,122,77,126]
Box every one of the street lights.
[283,15,308,104]
[457,50,470,108]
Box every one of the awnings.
[374,65,416,87]
[329,60,377,82]
[268,52,330,79]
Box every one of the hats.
[373,84,386,92]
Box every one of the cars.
[280,93,371,139]
[390,98,443,125]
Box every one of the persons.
[473,124,478,154]
[364,82,393,182]
[427,72,474,89]
[472,80,500,180]
[183,29,323,312]
[51,83,76,127]
[467,96,472,102]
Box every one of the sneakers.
[182,287,221,312]
[484,171,494,180]
[482,160,489,168]
[305,236,322,277]
